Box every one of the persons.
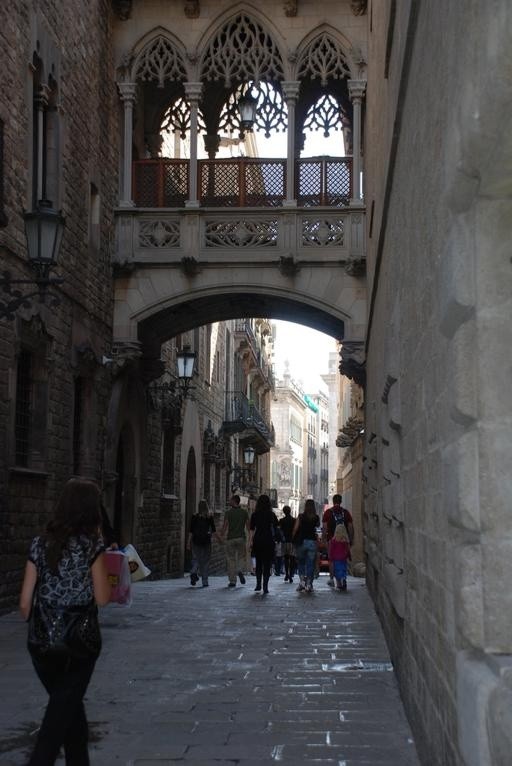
[189,494,354,594]
[19,478,116,765]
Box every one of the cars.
[316,530,328,570]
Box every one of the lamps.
[145,341,201,394]
[235,75,259,128]
[232,445,256,474]
[0,197,72,286]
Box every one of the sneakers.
[191,568,346,593]
